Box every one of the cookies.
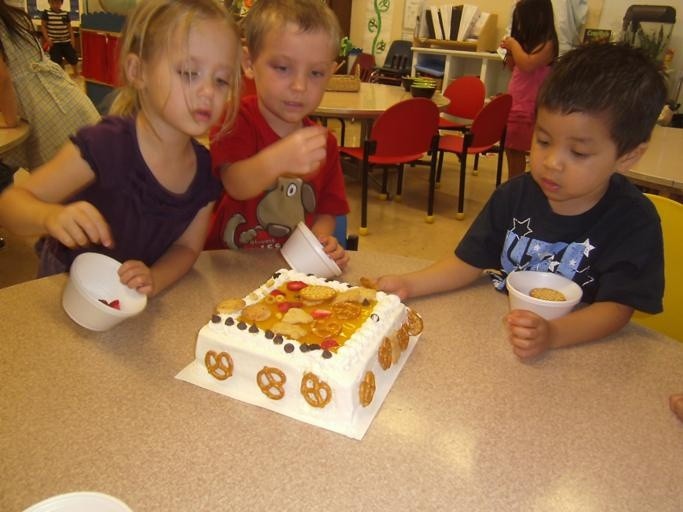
[530,288,566,301]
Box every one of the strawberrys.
[100,300,119,308]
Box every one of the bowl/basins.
[506,271,582,318]
[280,221,341,281]
[400,74,437,98]
[62,249,148,330]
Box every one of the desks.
[1,120,31,151]
[614,127,683,199]
[0,246,683,512]
[308,82,451,199]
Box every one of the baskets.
[326,64,360,92]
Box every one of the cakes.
[195,268,423,424]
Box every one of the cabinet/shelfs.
[410,47,512,145]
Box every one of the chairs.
[411,76,485,188]
[630,194,683,343]
[338,98,439,235]
[435,94,512,218]
[369,40,413,86]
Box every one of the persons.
[210,0,351,271]
[0,0,103,172]
[0,0,243,299]
[500,0,559,180]
[41,0,79,77]
[669,394,683,423]
[376,41,668,359]
